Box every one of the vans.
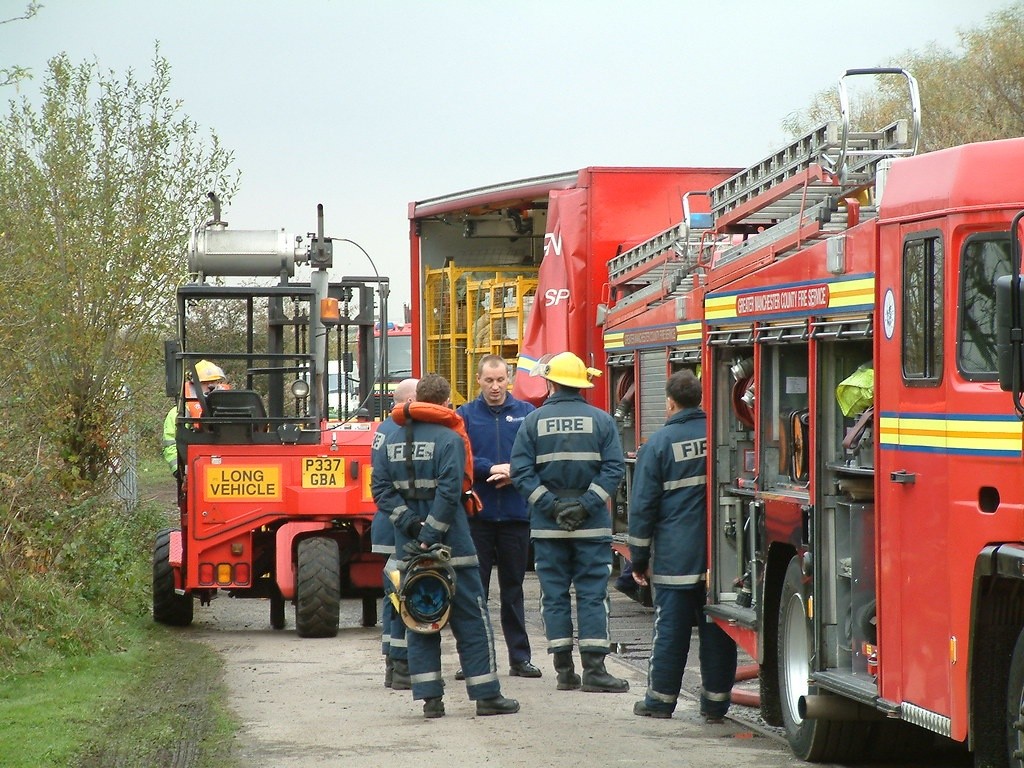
[297,361,360,416]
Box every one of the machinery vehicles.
[151,191,392,638]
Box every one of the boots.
[509,658,542,678]
[700,709,724,724]
[553,651,581,690]
[476,695,520,716]
[581,651,630,693]
[455,668,465,680]
[423,698,446,719]
[385,656,445,690]
[633,701,672,719]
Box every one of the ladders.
[606,64,923,298]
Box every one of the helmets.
[190,359,227,382]
[399,553,457,635]
[540,351,595,389]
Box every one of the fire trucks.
[409,166,744,570]
[603,67,923,570]
[703,138,1022,768]
[342,323,413,423]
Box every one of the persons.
[510,351,629,693]
[452,354,543,677]
[163,360,234,478]
[370,373,520,718]
[370,379,445,690]
[628,369,737,724]
